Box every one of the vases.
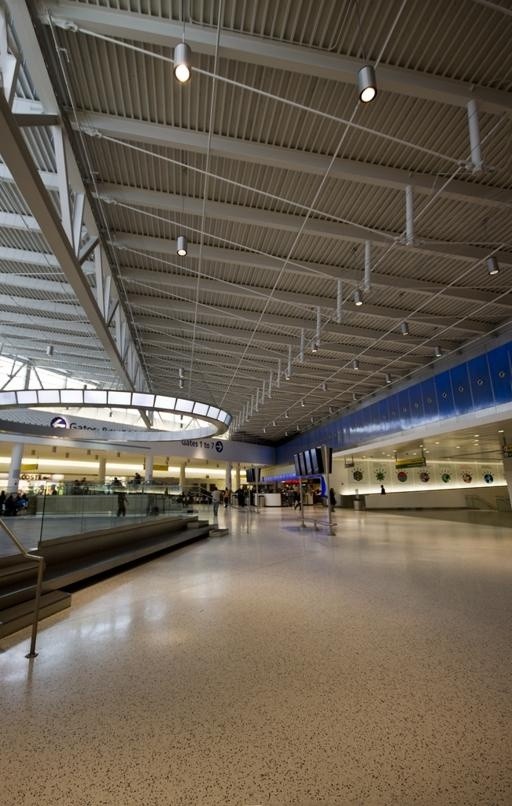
[352,498,362,510]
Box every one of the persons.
[0,470,336,517]
[379,484,386,495]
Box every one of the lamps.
[176,167,188,256]
[173,0,194,82]
[47,328,54,356]
[178,358,185,389]
[178,344,186,378]
